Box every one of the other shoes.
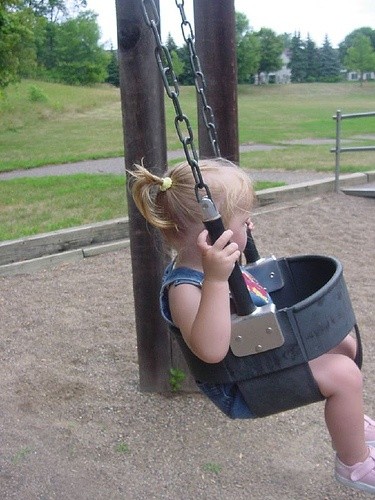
[335,442,375,496]
[363,413,375,446]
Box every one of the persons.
[126,157,375,495]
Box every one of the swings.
[114,1,364,422]
